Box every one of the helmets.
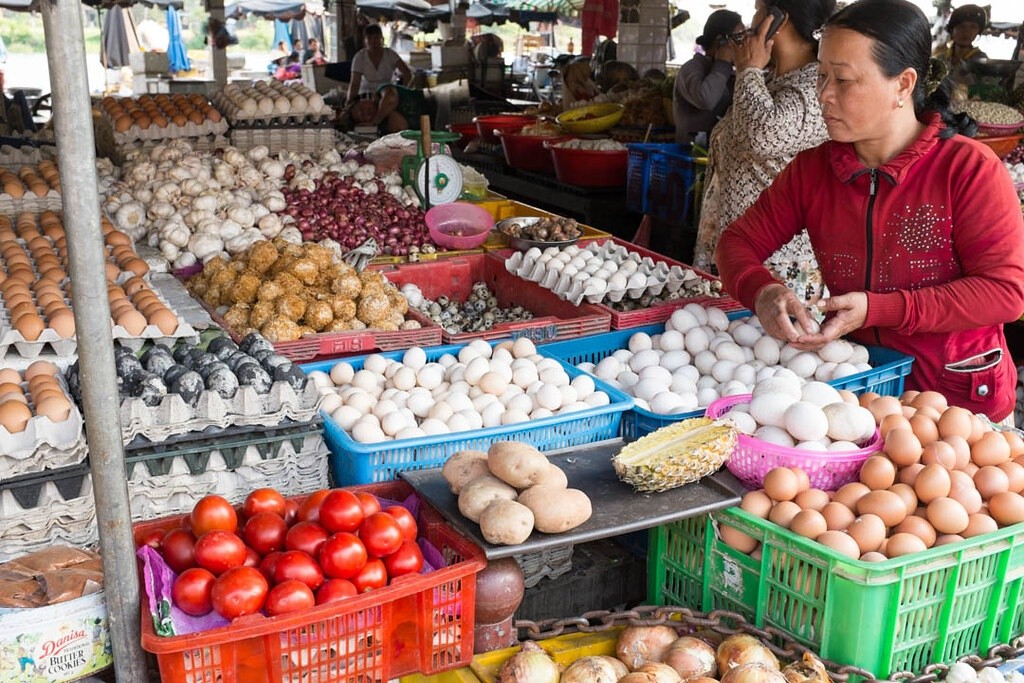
[948,4,986,34]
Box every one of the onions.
[282,164,431,256]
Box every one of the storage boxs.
[294,338,633,485]
[535,312,915,440]
[129,52,216,100]
[624,142,695,221]
[397,46,470,104]
[646,505,1023,683]
[131,480,486,683]
[299,63,348,95]
[0,592,114,682]
[167,193,746,366]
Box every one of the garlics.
[103,140,419,272]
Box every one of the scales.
[400,130,463,208]
[964,58,1024,102]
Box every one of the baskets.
[1,76,1024,683]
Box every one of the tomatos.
[158,487,424,622]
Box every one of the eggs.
[101,80,325,131]
[1,155,307,457]
[309,237,1024,676]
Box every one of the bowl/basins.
[424,203,584,250]
[444,103,626,187]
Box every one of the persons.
[715,0,1024,432]
[672,10,748,146]
[279,38,326,65]
[691,0,842,307]
[344,25,415,136]
